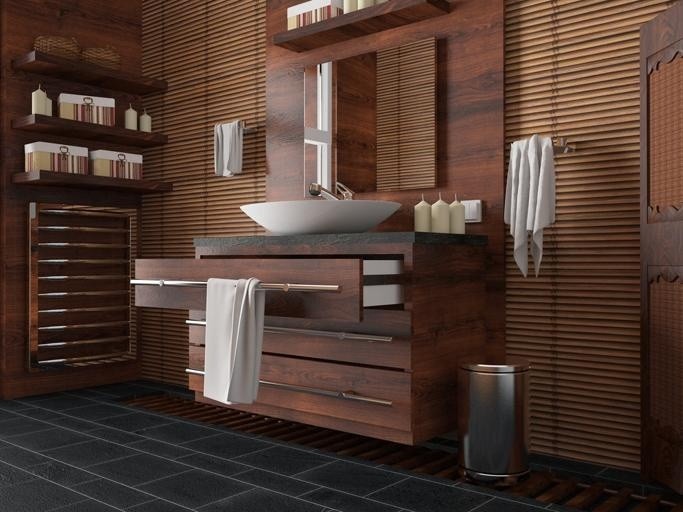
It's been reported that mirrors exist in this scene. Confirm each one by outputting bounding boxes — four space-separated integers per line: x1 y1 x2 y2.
304 37 436 193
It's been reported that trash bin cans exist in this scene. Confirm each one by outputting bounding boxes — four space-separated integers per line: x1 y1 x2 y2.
457 355 532 487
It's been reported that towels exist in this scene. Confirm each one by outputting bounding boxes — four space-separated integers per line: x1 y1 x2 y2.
213 120 244 178
503 135 556 279
202 277 265 407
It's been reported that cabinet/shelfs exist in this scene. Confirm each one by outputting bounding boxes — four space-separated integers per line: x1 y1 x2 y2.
133 232 503 447
10 50 175 192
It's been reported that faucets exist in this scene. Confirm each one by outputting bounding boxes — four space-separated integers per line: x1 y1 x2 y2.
308 181 355 200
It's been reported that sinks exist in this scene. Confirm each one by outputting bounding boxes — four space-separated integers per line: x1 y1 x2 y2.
239 199 403 234
